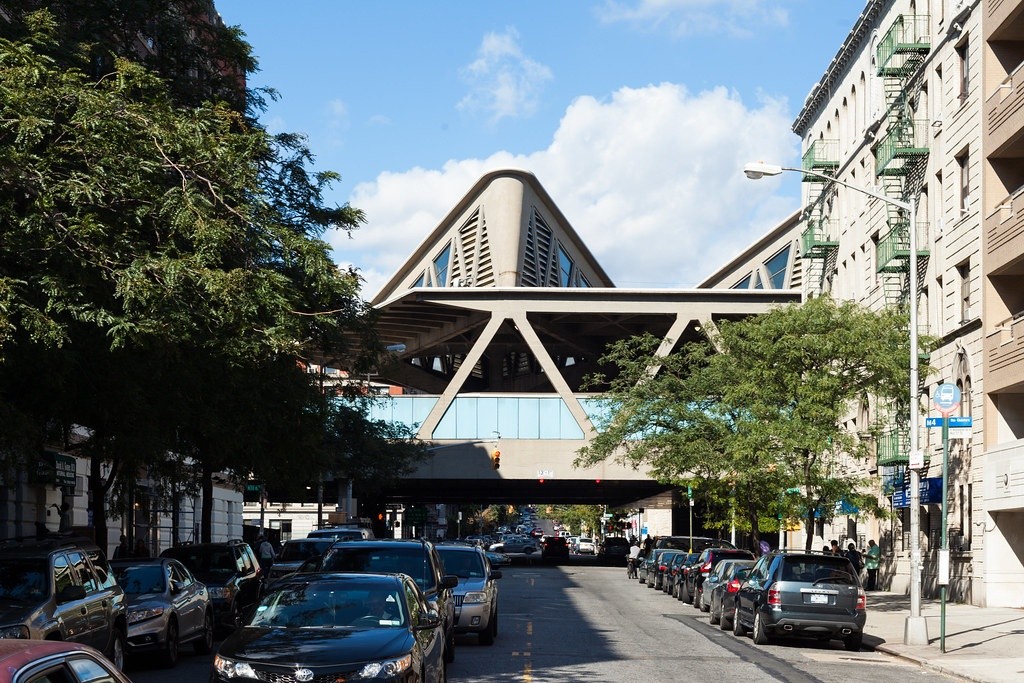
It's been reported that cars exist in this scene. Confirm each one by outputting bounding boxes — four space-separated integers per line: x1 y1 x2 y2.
0 638 133 683
638 535 756 608
489 509 569 558
599 537 629 559
434 544 503 645
108 558 216 667
699 559 755 612
441 535 511 570
709 562 757 631
209 571 447 683
269 539 338 586
552 519 595 555
307 528 375 543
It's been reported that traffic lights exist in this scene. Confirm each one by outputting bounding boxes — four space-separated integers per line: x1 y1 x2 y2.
491 450 500 470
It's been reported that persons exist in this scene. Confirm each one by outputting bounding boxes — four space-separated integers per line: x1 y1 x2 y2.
471 541 490 551
52 503 73 532
628 534 652 579
823 540 880 591
254 535 276 579
134 539 150 559
113 535 133 559
420 532 447 544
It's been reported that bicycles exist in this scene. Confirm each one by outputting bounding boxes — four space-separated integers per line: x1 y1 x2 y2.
625 554 634 579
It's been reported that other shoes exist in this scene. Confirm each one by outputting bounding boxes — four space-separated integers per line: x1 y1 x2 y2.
864 588 874 591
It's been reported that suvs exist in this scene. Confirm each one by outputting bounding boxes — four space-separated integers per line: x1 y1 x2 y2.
143 538 266 632
315 534 459 662
733 548 867 651
0 533 128 672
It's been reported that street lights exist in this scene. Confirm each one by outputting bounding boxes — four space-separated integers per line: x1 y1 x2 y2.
745 161 929 645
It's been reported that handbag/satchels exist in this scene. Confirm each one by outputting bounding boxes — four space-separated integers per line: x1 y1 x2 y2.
857 552 865 570
